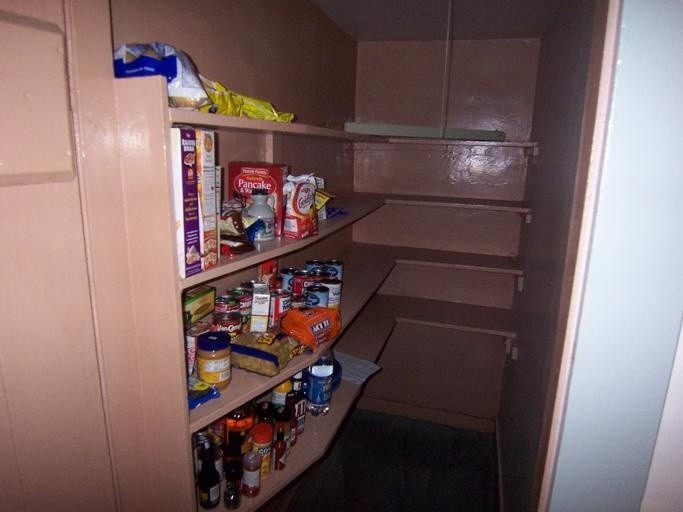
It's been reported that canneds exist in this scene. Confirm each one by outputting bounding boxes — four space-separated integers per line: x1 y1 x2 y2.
213 259 345 334
196 331 234 391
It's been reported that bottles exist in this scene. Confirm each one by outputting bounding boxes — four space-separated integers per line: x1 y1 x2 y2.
306 348 334 416
246 188 277 241
223 372 309 512
198 437 221 509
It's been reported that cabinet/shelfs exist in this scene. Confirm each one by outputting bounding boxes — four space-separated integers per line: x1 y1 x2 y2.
120 106 538 512
